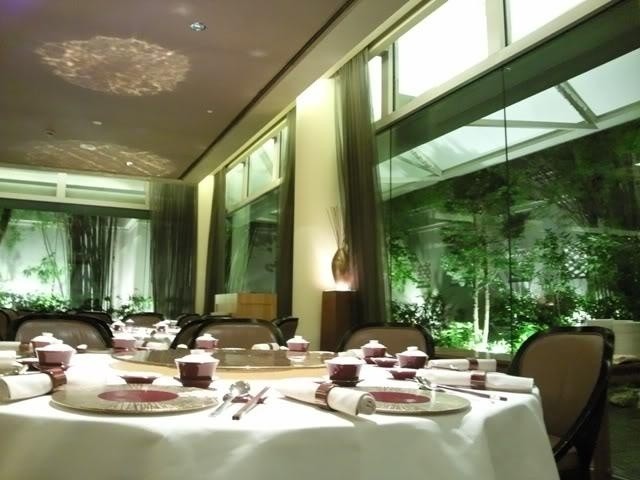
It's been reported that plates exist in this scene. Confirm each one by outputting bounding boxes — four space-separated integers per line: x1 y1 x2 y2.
32 363 73 373
120 375 159 384
338 385 471 415
372 357 397 366
51 383 223 417
173 376 219 389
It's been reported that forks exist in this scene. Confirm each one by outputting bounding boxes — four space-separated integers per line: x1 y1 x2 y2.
415 375 445 393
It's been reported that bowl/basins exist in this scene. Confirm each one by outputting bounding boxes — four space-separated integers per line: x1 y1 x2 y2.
386 369 416 380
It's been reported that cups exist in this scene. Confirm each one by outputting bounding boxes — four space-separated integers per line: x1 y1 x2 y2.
30 332 77 365
174 349 221 380
111 332 137 348
397 345 427 368
194 333 219 349
111 318 178 333
286 335 311 351
360 340 387 364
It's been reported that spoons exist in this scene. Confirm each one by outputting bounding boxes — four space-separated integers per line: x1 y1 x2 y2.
210 381 250 418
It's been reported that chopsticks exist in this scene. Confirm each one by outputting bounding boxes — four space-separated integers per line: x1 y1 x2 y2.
232 384 272 419
438 385 508 401
